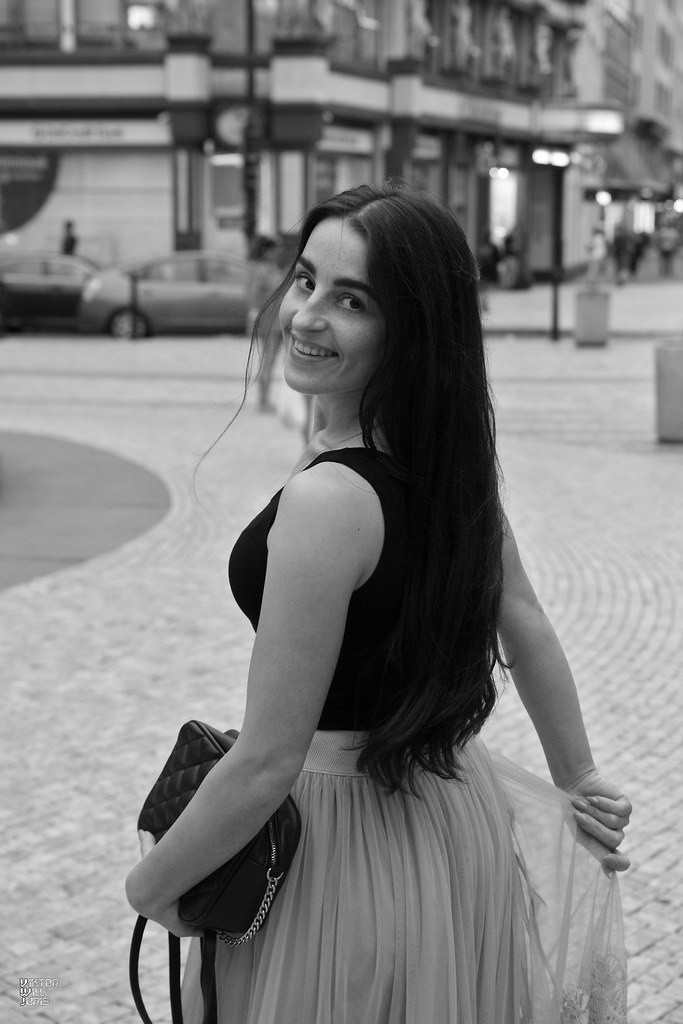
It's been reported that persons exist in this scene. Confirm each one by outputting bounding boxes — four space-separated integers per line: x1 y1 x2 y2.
57 219 78 255
126 181 641 1024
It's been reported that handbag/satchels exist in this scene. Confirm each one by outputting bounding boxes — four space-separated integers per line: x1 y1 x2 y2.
138 718 301 948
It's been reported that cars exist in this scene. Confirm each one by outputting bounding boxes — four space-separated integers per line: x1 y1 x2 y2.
80 251 286 340
1 251 106 328
